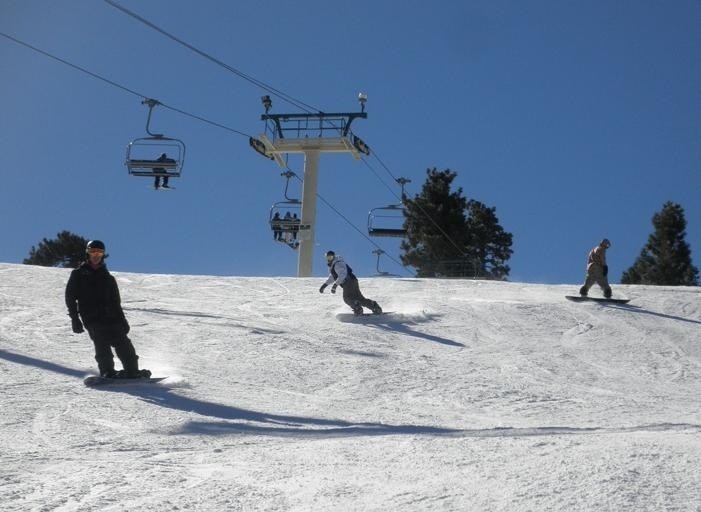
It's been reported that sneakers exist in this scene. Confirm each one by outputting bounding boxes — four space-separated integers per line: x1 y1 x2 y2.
354 308 363 315
371 301 382 314
102 370 151 378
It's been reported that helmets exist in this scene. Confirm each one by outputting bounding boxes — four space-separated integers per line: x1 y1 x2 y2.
85 240 105 260
327 251 334 256
600 239 611 249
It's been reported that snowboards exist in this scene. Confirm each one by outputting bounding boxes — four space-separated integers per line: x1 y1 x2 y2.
565 296 630 304
336 312 403 325
84 374 167 386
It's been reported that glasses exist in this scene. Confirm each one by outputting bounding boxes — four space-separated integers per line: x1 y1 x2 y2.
88 249 104 256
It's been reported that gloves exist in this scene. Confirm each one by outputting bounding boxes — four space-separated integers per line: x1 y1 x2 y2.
331 284 337 293
603 265 608 276
123 318 129 334
320 284 328 293
72 319 84 333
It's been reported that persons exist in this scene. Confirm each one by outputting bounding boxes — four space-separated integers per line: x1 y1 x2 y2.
151 151 178 190
578 237 614 300
282 211 294 242
318 247 386 318
293 214 301 241
64 238 151 386
273 212 284 242
